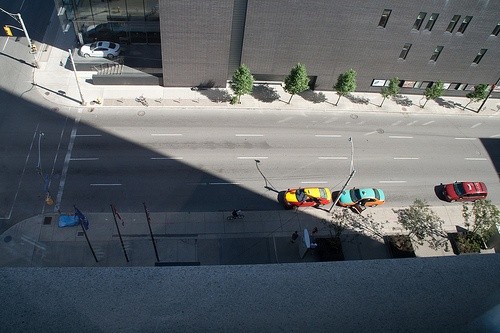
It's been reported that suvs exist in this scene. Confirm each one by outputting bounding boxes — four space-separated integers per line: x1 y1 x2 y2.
442 181 488 203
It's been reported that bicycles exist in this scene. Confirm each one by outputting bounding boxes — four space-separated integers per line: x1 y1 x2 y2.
226 210 245 222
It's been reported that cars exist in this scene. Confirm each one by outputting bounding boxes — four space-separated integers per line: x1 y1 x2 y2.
80 41 121 59
336 187 385 208
282 187 332 209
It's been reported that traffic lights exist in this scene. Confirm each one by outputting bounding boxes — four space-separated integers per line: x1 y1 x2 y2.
4 27 12 36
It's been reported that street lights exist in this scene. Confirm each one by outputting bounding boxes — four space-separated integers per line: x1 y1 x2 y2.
328 137 357 214
34 132 61 214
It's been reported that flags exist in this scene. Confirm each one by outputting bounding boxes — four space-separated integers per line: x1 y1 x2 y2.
77 209 89 230
47 194 54 206
113 208 124 227
145 206 151 221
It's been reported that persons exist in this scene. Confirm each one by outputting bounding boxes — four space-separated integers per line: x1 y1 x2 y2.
232 209 239 219
312 226 318 237
291 231 299 244
